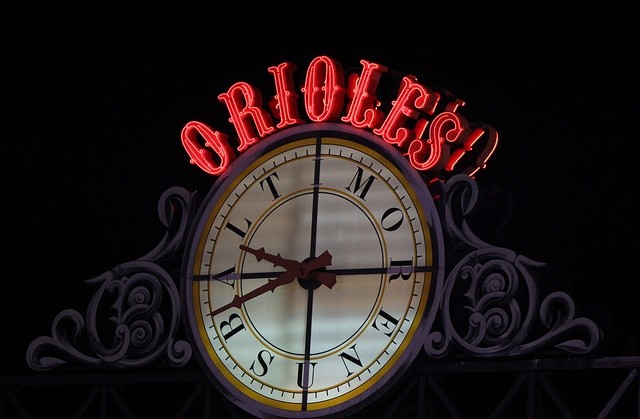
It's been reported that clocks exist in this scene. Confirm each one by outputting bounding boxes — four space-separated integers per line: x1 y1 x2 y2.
183 122 445 419
